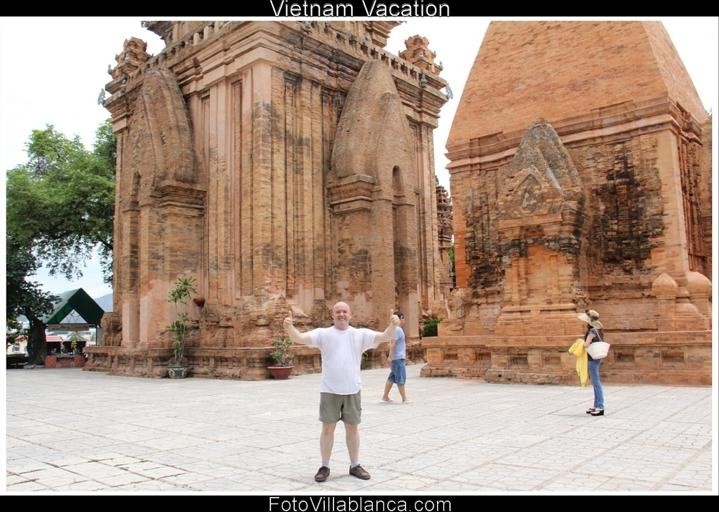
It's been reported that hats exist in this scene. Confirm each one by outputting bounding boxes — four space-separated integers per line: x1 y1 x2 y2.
578 310 604 329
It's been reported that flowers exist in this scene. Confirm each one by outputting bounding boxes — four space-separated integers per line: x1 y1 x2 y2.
265 329 296 366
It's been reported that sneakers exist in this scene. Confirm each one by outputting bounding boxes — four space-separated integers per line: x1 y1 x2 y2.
403 400 414 404
314 466 330 482
381 399 393 404
350 464 370 479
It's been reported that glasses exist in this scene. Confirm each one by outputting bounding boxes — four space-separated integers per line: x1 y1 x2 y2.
401 317 404 320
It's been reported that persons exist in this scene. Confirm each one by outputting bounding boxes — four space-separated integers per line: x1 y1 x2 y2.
283 302 402 482
577 310 604 416
380 311 414 405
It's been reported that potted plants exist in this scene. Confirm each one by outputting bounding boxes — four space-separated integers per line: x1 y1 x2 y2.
164 275 201 378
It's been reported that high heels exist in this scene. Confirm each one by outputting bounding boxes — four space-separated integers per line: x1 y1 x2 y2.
586 408 595 414
591 410 604 415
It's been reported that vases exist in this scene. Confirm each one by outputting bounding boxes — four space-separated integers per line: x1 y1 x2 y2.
267 366 293 378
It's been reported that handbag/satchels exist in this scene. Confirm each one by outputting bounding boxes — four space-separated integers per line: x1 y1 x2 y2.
585 342 610 359
